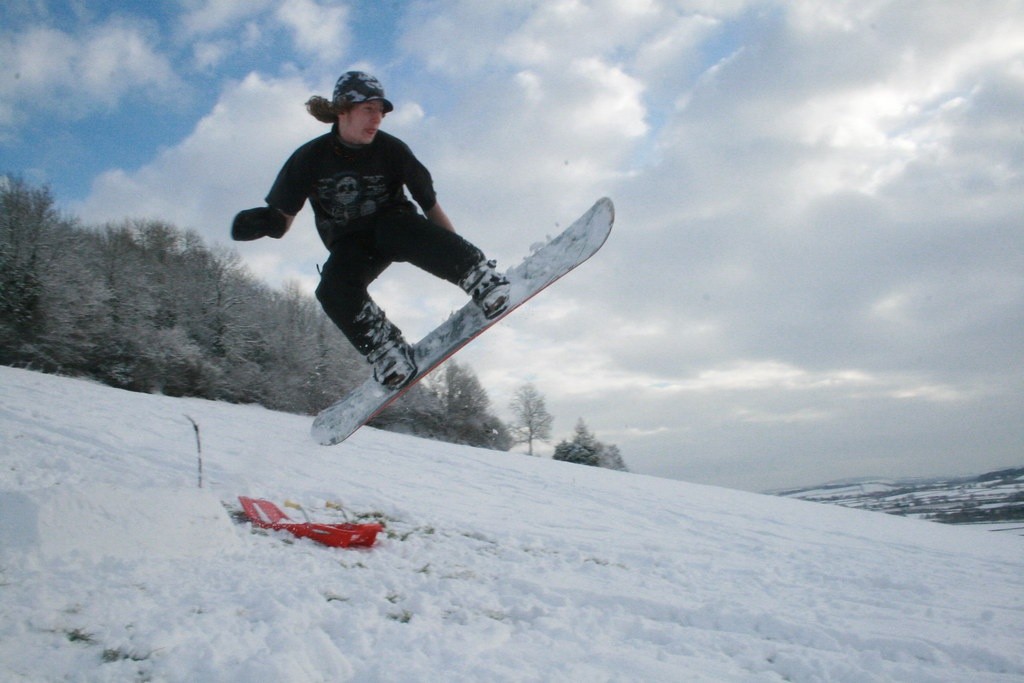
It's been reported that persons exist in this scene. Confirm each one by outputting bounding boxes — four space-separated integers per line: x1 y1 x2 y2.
231 68 512 392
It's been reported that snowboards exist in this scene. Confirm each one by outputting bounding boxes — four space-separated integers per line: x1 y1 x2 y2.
310 197 613 448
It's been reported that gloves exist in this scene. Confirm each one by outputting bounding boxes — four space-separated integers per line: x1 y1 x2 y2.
232 205 288 241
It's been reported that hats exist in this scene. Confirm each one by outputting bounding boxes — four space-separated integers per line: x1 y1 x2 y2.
333 71 393 113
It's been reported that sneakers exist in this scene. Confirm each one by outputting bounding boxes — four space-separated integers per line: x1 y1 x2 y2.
367 338 417 390
459 260 510 319
